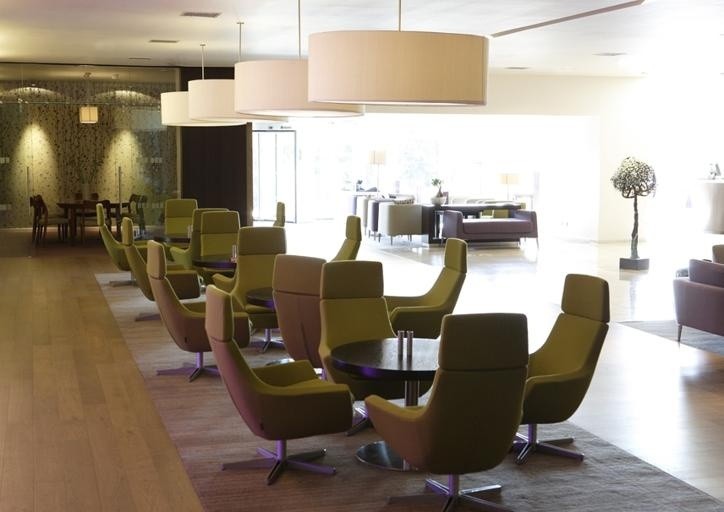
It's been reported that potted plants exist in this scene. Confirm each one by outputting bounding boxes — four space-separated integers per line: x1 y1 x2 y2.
429 178 446 205
610 156 657 270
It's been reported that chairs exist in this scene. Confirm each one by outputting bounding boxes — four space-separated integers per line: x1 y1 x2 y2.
29 192 150 247
673 244 723 344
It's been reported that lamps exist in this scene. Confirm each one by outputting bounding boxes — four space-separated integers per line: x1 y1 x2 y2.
78 72 97 125
